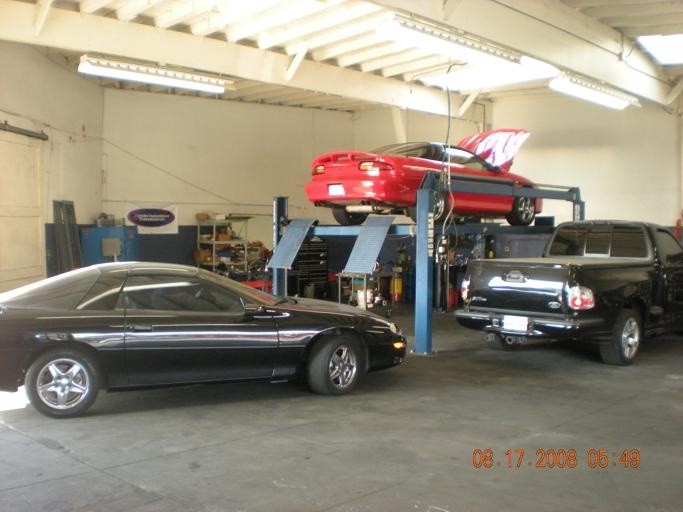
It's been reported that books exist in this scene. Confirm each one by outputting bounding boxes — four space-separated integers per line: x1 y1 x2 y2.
207 213 225 220
219 256 231 263
228 213 253 219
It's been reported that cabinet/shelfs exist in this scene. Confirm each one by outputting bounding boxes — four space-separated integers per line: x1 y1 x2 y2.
196 221 249 274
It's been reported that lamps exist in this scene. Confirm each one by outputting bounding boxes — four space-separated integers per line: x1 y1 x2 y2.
78 55 234 95
551 72 638 114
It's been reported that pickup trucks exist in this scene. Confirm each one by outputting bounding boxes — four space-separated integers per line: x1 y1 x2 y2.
454 220 683 366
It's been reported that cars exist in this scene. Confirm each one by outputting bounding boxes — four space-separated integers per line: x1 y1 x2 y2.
0 262 407 419
305 129 543 224
